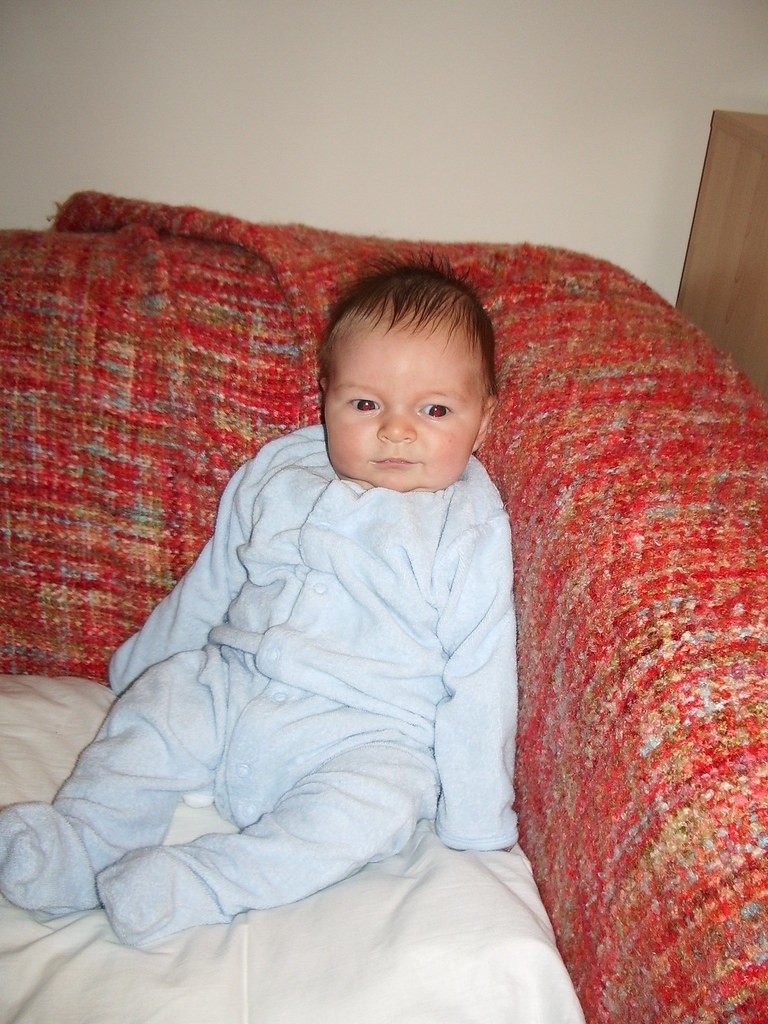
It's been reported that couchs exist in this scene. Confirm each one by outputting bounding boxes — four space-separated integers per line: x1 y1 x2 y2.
0 190 768 1024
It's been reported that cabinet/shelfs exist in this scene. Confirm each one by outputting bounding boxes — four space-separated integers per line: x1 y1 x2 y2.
676 109 768 399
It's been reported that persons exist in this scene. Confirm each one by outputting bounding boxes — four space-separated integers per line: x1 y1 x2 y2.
0 254 518 944
